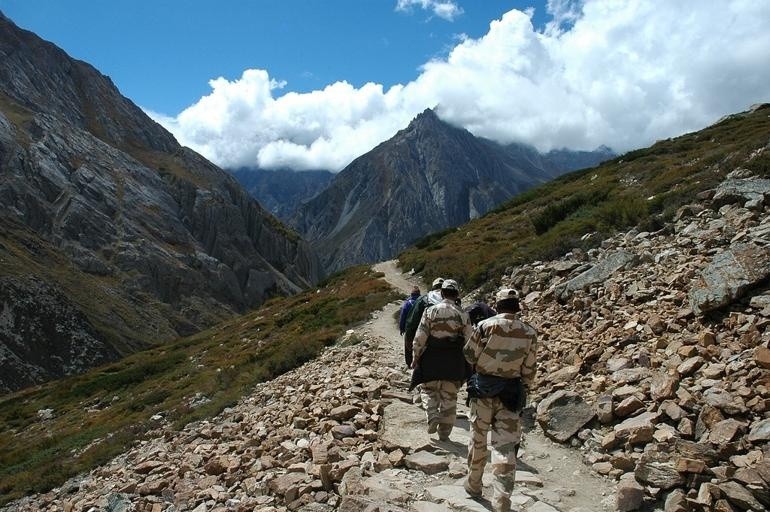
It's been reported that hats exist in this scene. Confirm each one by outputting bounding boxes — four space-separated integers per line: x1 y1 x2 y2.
442 279 458 291
432 278 444 286
496 289 519 302
411 286 420 294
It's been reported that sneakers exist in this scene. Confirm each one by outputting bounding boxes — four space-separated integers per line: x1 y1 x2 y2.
463 479 483 499
427 422 449 441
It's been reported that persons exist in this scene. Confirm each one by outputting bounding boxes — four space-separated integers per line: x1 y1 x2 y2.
408 279 472 442
462 286 539 511
465 292 498 408
404 277 446 353
399 285 421 370
